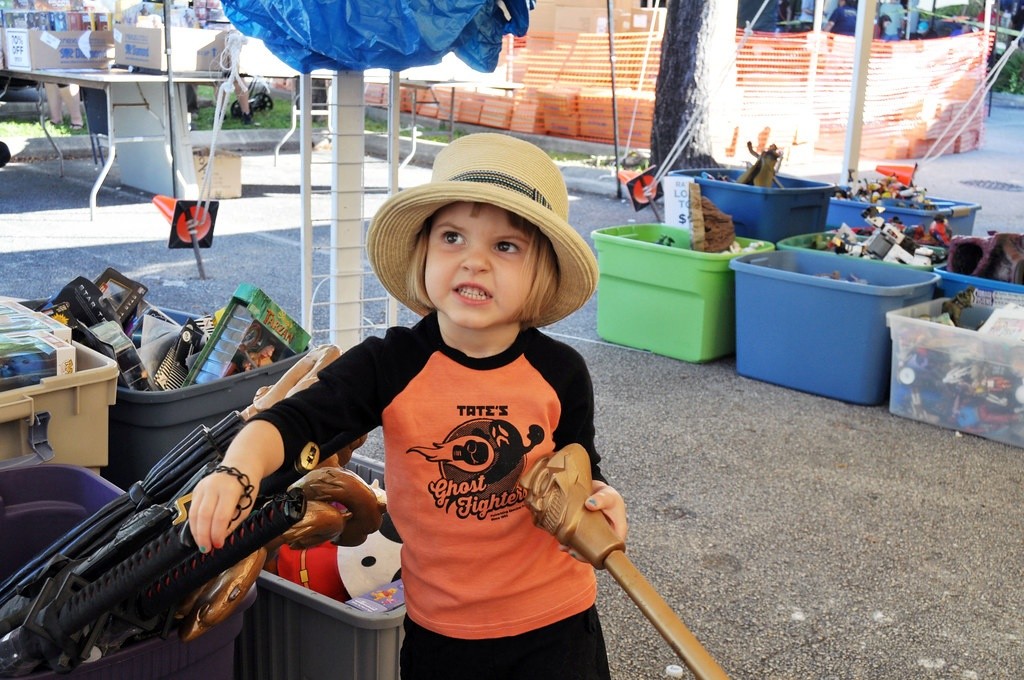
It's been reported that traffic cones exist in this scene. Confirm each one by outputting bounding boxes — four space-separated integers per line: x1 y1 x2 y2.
152 195 220 249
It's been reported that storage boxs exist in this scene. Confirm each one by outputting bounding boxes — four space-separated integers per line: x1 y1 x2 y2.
192 145 243 198
777 231 949 298
0 463 258 680
669 167 836 245
589 223 775 363
0 300 77 393
113 23 229 73
0 340 122 474
2 28 114 70
100 344 312 493
76 319 158 392
727 250 942 404
232 452 407 680
934 263 1024 327
825 185 982 235
180 283 313 385
884 297 1024 449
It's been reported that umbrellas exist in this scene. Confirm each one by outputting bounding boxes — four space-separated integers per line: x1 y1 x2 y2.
0 345 341 604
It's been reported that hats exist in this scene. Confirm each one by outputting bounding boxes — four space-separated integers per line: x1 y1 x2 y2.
365 133 599 328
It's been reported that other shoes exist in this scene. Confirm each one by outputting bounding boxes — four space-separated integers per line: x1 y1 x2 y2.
212 112 226 122
240 111 253 124
192 111 200 120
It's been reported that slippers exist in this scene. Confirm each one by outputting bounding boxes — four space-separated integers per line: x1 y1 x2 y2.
70 127 84 131
50 121 64 125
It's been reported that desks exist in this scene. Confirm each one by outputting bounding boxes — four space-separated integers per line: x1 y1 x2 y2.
0 68 273 229
275 75 526 180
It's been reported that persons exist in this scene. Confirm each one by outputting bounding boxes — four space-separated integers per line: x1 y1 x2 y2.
188 130 625 680
44 83 86 130
214 80 254 124
184 83 199 121
776 0 1024 50
